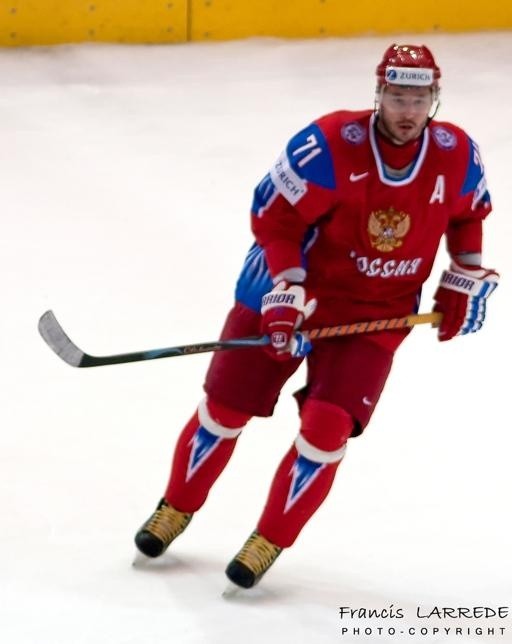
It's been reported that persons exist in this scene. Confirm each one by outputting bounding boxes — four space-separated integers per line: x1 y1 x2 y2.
135 43 499 589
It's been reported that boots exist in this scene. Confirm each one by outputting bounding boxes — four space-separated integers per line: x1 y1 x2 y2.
225 530 283 589
134 497 194 558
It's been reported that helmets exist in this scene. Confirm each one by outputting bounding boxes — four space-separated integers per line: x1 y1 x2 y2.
376 44 441 88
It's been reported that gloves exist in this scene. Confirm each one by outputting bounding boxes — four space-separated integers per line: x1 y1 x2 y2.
259 280 312 363
432 256 501 341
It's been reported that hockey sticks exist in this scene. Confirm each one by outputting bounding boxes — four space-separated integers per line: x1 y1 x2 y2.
37 310 446 368
